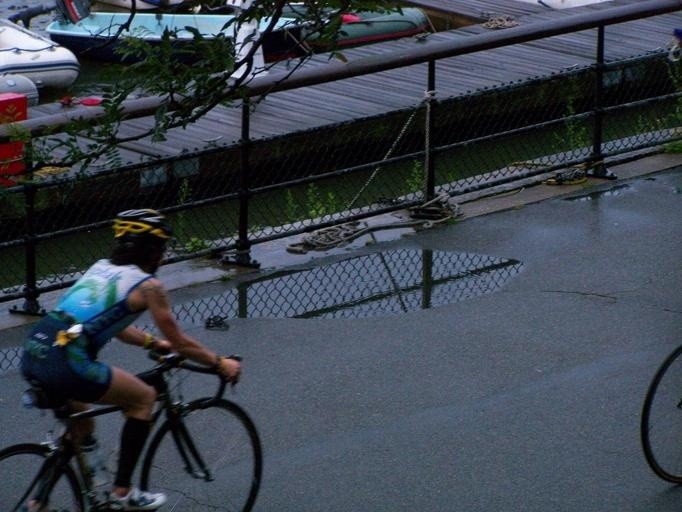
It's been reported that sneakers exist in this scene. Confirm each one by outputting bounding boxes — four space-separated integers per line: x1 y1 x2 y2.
81 470 166 512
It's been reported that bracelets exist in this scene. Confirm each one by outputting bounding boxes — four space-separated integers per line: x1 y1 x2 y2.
142 333 155 350
213 354 226 373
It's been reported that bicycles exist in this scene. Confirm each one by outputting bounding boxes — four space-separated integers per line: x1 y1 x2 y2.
0 347 263 512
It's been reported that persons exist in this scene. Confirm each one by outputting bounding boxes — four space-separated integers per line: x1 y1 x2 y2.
18 208 243 512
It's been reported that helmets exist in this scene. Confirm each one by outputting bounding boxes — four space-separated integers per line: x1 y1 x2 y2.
114 208 172 244
